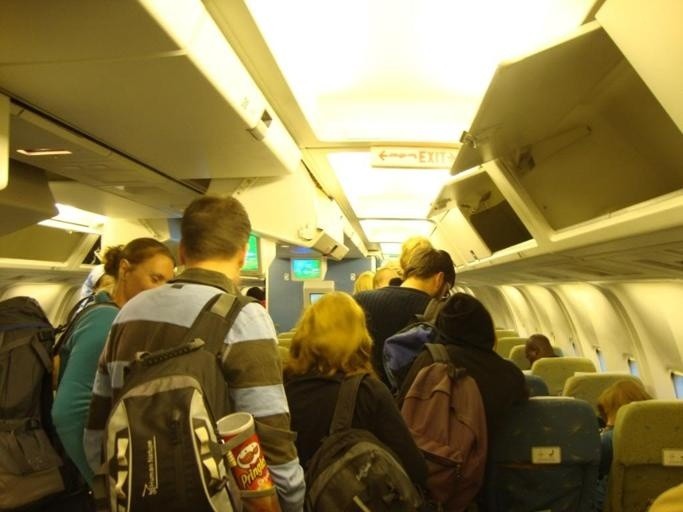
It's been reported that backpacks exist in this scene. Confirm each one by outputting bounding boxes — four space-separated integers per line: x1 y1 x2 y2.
303 427 437 512
399 345 488 510
381 296 447 393
0 294 119 512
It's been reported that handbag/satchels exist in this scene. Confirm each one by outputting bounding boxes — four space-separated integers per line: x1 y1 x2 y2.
104 292 274 512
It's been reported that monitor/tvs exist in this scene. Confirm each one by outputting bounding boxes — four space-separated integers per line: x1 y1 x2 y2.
290 255 328 281
239 232 276 279
310 292 325 305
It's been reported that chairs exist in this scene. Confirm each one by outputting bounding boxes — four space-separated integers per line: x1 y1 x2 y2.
486 330 683 512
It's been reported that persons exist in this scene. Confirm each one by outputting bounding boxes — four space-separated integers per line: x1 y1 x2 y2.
525 335 558 368
280 237 525 510
597 379 653 479
48 196 305 512
